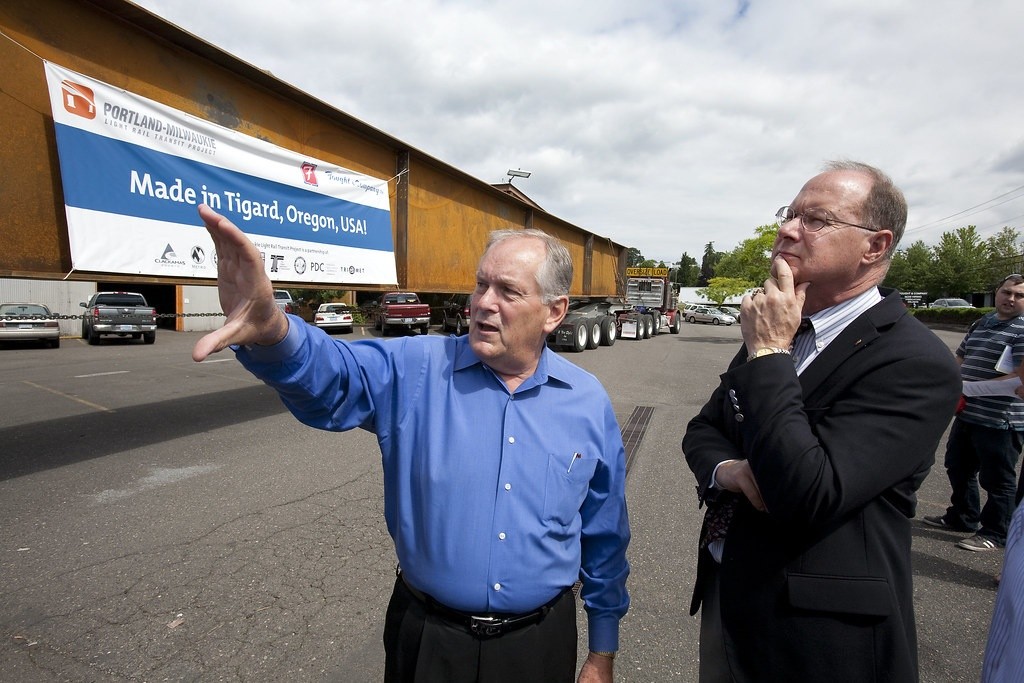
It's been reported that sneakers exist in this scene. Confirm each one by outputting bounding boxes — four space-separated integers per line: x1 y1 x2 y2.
923 515 978 533
957 533 1000 550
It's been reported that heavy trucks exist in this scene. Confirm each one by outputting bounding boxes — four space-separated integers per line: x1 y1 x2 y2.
544 266 681 353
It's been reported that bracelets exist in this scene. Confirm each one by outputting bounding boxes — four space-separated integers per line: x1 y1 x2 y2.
590 650 616 659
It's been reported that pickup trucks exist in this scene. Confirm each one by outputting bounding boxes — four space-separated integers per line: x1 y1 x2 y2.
79 292 157 344
373 293 430 336
273 290 303 314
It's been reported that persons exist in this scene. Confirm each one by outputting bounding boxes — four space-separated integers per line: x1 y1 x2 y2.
976 365 1023 682
924 273 1024 552
191 204 632 683
682 160 962 683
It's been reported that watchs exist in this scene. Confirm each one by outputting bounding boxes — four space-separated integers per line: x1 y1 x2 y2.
746 347 790 363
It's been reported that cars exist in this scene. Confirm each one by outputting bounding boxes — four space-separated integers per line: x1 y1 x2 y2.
0 302 61 349
905 302 933 308
930 299 977 309
312 303 354 333
716 307 740 322
685 308 736 326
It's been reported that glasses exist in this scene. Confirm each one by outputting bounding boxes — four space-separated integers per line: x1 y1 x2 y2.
1004 273 1024 281
774 206 876 235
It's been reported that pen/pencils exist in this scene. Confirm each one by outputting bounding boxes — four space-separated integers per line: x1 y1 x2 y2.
568 452 582 473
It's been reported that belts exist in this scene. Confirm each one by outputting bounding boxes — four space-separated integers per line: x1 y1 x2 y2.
396 572 575 637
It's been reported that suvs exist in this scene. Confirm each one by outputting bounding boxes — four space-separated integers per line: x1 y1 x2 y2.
442 293 471 336
682 304 710 317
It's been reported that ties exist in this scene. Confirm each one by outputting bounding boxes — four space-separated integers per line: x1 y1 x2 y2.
699 317 812 551
